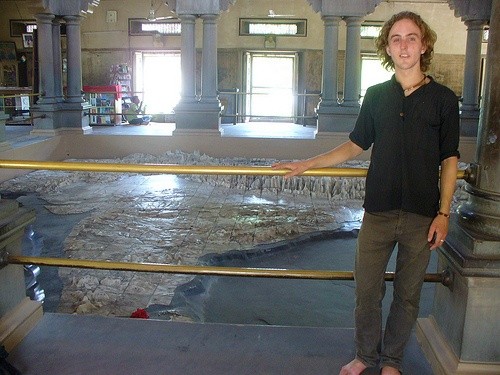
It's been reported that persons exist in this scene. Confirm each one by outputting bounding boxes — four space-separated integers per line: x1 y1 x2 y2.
126 96 149 125
271 11 460 375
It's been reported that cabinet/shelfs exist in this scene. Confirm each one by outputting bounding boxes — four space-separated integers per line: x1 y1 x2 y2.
82 85 122 126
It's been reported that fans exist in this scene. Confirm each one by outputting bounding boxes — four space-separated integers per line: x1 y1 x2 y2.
248 0 296 18
132 0 177 23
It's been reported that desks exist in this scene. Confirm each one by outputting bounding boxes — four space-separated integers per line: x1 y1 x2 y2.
218 88 240 125
302 88 343 126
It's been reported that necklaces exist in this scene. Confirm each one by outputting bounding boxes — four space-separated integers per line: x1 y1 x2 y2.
403 72 426 92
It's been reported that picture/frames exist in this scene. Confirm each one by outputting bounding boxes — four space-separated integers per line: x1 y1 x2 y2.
21 33 34 49
0 41 18 61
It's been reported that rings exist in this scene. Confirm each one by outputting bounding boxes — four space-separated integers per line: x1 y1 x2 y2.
440 240 444 242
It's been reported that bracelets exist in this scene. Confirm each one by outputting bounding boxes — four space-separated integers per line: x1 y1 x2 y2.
438 211 451 219
